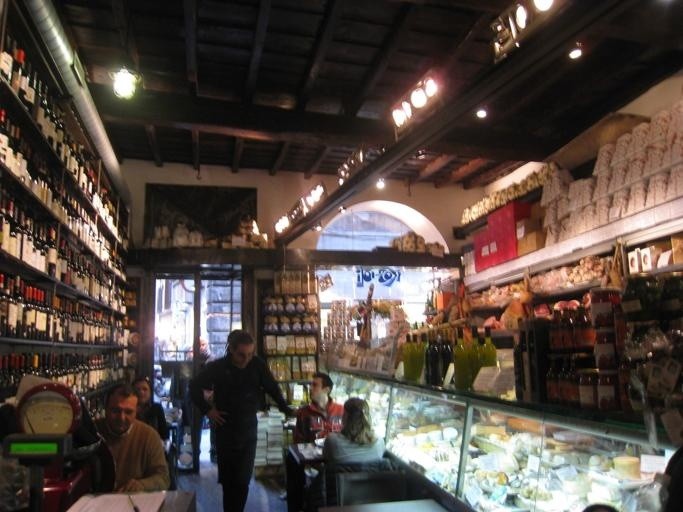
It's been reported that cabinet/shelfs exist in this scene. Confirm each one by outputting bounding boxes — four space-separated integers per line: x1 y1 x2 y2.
0 1 140 433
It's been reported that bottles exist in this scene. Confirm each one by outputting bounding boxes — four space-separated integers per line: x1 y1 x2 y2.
513 330 532 402
0 32 129 419
545 356 579 408
547 306 596 355
401 324 498 392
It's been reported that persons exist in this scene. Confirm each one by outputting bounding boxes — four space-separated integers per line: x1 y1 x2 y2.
153 368 170 405
189 331 293 511
87 383 172 495
130 379 168 442
295 371 344 440
197 335 215 364
322 396 384 464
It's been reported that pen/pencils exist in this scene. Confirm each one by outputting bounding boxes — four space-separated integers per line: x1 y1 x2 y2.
128 496 140 512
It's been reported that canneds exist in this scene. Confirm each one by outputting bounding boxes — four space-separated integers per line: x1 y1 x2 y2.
577 263 683 412
